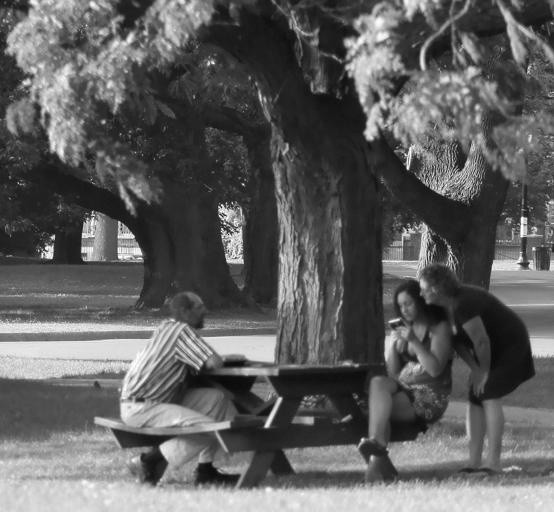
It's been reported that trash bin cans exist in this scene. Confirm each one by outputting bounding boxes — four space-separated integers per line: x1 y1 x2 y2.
531 246 550 271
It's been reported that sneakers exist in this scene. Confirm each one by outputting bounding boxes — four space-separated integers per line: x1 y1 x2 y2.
126 454 154 486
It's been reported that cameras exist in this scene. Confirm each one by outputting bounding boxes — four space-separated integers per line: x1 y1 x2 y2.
388 319 407 331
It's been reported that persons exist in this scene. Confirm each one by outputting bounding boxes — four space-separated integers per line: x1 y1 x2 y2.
356 280 459 481
420 264 535 477
119 292 242 486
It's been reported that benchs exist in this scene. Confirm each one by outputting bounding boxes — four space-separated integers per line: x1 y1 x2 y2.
93 414 269 436
294 407 434 432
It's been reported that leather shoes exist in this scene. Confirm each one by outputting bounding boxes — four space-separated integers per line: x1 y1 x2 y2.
193 469 241 487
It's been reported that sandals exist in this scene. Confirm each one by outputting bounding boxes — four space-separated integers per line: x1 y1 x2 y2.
358 438 388 458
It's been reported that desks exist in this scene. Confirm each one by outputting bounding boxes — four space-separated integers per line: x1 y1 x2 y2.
189 365 399 490
